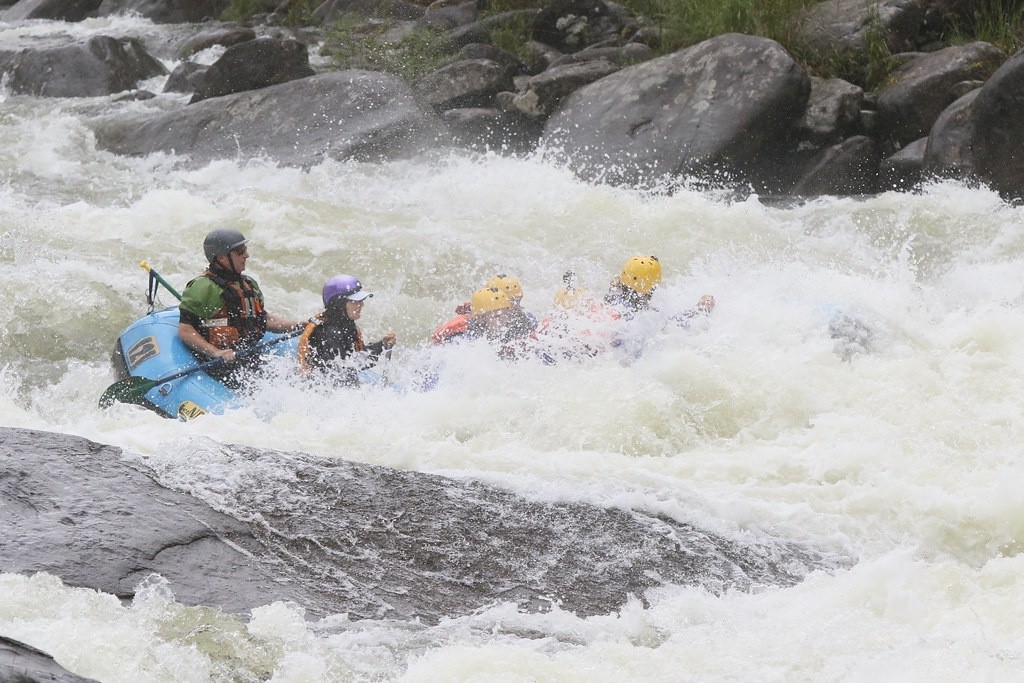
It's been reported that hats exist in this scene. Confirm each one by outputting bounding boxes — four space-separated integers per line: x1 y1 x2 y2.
344 291 373 304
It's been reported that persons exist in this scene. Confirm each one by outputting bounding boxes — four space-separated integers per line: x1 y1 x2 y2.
296 274 396 387
433 256 714 357
178 227 311 391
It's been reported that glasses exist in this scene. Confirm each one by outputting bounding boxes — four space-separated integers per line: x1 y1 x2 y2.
233 246 247 254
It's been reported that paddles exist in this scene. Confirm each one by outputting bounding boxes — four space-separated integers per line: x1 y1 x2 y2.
98 316 310 415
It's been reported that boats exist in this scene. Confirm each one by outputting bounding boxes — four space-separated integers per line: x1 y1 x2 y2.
112 305 304 421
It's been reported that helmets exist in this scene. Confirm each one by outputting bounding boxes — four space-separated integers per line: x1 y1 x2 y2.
620 256 662 293
203 229 248 265
321 274 361 309
470 288 510 317
485 274 523 299
